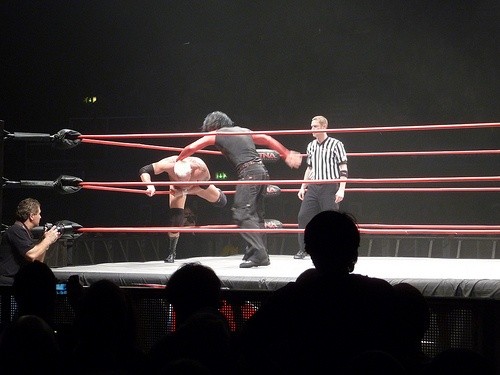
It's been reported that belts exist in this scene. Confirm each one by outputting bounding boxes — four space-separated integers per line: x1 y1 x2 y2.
237 160 263 170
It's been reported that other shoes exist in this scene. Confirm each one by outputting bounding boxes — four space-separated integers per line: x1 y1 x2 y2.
163 248 178 264
293 249 308 260
238 255 270 269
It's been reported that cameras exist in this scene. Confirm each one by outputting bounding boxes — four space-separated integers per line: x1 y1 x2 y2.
56 284 68 297
44 222 72 239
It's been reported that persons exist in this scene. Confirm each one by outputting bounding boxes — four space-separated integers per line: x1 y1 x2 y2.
176 207 201 260
0 198 63 337
295 210 393 374
294 116 348 259
174 111 302 268
139 155 227 263
0 262 489 375
